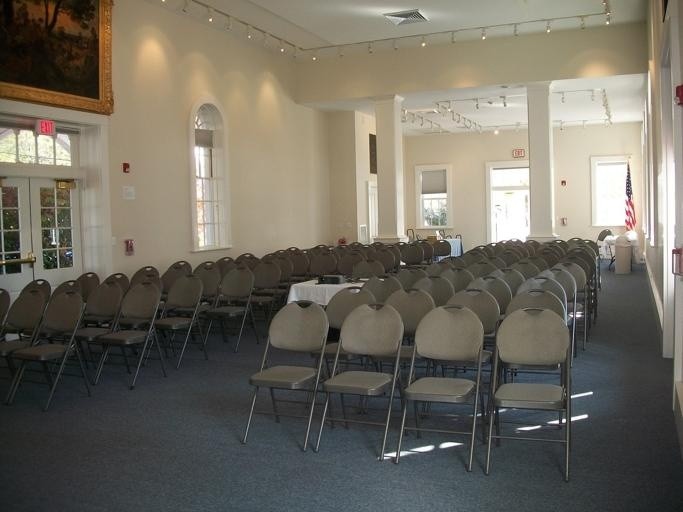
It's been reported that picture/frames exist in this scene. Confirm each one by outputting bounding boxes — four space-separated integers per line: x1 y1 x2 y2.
0 0 115 115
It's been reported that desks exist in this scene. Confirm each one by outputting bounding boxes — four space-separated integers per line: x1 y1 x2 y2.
606 241 640 271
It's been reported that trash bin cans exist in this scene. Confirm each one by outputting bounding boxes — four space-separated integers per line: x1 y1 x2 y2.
614 236 633 275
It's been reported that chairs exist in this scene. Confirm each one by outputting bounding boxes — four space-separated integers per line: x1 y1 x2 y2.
596 229 612 261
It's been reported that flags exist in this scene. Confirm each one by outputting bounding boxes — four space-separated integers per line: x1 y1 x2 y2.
625 161 637 231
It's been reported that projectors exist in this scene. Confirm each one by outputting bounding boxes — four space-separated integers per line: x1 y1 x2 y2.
318 274 344 283
